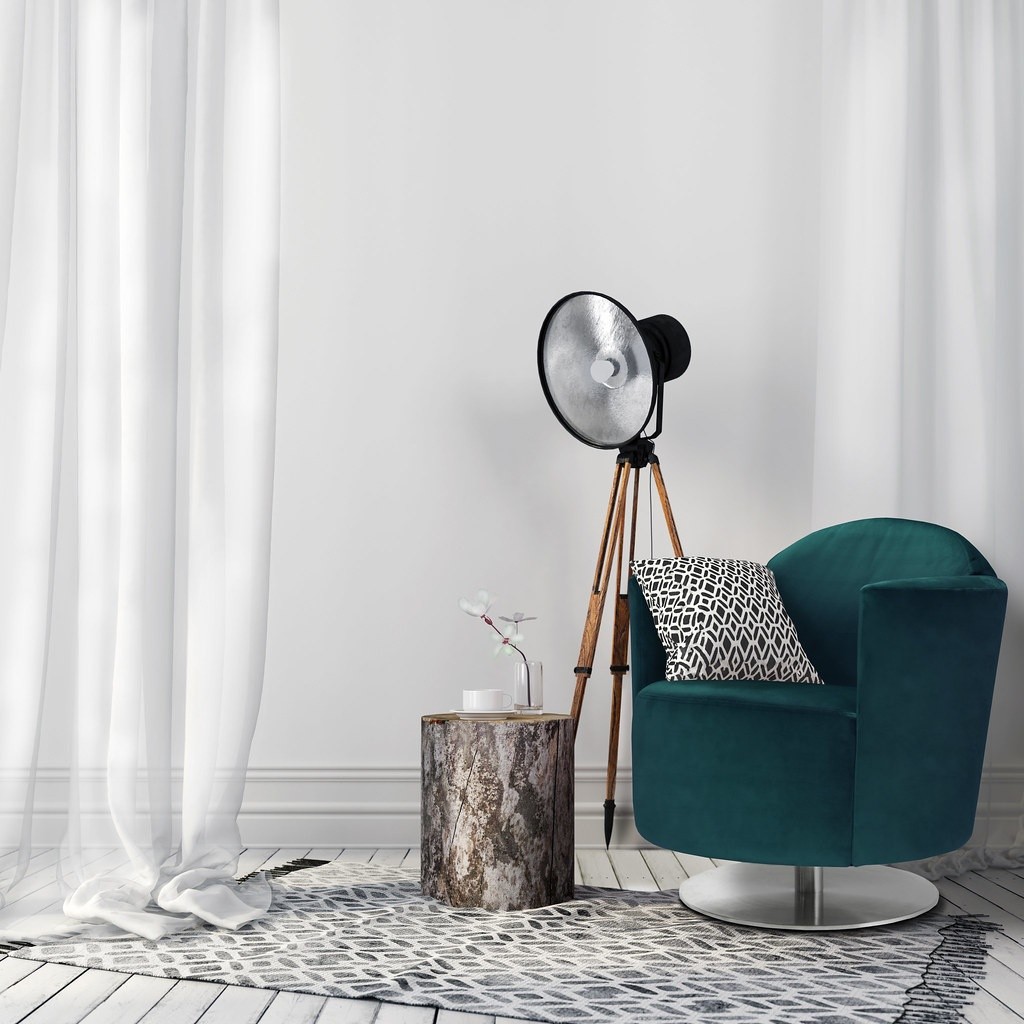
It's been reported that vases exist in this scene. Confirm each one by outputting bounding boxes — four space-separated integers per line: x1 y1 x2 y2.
514 658 546 716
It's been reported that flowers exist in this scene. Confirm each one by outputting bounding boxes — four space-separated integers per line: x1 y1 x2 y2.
459 589 539 706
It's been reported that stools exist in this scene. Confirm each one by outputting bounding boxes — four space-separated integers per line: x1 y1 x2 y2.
420 713 576 911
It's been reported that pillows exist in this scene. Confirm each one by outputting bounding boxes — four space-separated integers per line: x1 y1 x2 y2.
629 558 826 686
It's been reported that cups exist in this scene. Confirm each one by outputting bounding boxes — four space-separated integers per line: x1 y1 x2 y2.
463 689 513 711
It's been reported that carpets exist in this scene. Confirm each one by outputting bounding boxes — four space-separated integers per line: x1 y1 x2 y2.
0 857 1004 1024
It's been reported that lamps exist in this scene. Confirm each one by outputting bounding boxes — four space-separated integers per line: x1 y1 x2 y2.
536 291 694 468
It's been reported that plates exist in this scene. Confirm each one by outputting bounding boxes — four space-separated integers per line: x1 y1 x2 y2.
451 708 517 720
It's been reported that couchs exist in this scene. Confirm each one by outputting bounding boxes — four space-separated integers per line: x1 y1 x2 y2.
626 509 1012 937
